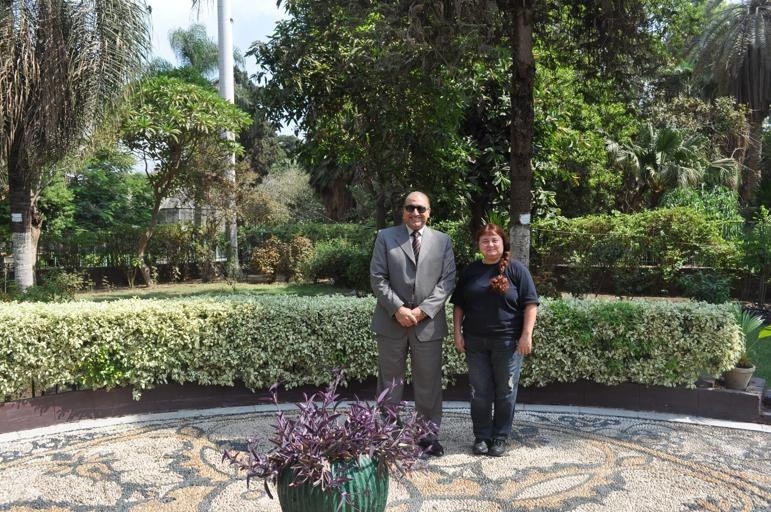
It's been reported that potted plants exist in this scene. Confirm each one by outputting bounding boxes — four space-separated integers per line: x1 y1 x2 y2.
208 364 442 512
720 296 771 391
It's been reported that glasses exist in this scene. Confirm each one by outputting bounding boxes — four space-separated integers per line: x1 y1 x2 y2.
403 204 431 214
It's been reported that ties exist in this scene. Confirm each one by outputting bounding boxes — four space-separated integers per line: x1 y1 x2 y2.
412 231 422 264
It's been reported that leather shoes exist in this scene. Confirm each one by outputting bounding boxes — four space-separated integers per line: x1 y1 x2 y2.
418 439 445 457
489 437 507 456
474 437 492 455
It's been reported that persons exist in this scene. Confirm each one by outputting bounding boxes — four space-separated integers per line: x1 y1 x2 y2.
449 221 542 457
367 191 459 458
